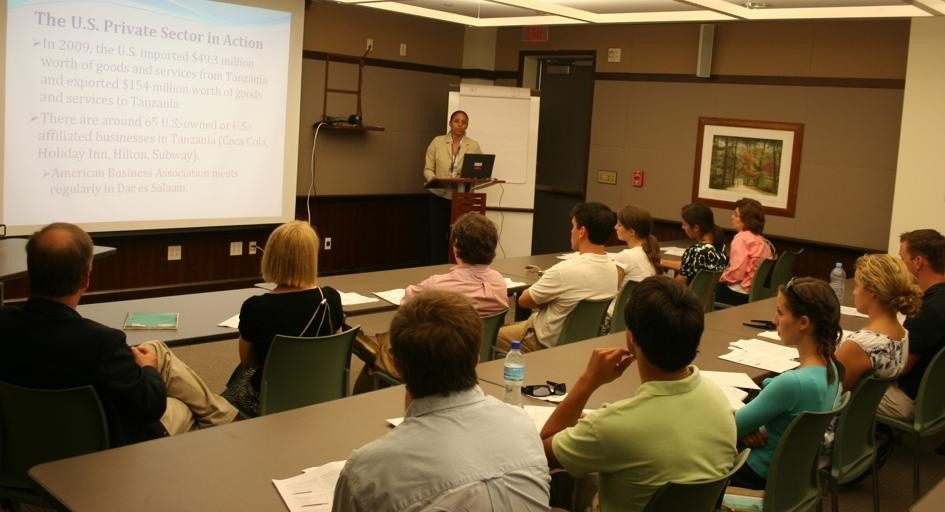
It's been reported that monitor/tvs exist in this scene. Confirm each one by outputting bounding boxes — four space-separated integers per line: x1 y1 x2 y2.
460 154 495 177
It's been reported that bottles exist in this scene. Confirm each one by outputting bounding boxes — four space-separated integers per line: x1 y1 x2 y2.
504 341 525 408
830 263 847 304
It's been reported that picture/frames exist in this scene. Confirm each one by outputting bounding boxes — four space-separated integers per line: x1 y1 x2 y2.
692 117 804 218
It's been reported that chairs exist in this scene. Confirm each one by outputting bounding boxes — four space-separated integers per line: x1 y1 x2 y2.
1 384 110 511
714 258 777 309
688 269 723 314
874 348 944 502
817 377 892 510
771 250 804 297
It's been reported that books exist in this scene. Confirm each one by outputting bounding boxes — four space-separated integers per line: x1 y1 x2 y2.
122 309 180 330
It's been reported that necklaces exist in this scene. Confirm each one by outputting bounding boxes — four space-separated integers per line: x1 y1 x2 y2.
794 355 820 370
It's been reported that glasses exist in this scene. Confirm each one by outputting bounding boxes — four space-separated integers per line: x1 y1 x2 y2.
522 381 566 396
787 277 800 301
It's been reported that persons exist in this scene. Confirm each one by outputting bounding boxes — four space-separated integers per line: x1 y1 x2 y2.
495 199 621 356
600 204 666 334
423 110 486 262
871 227 945 430
218 219 346 418
663 201 731 306
349 210 511 397
720 197 777 300
730 274 846 511
0 220 242 452
816 251 924 470
327 290 554 511
538 277 739 511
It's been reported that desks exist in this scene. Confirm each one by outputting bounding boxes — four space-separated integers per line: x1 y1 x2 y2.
74 238 696 366
27 279 871 511
